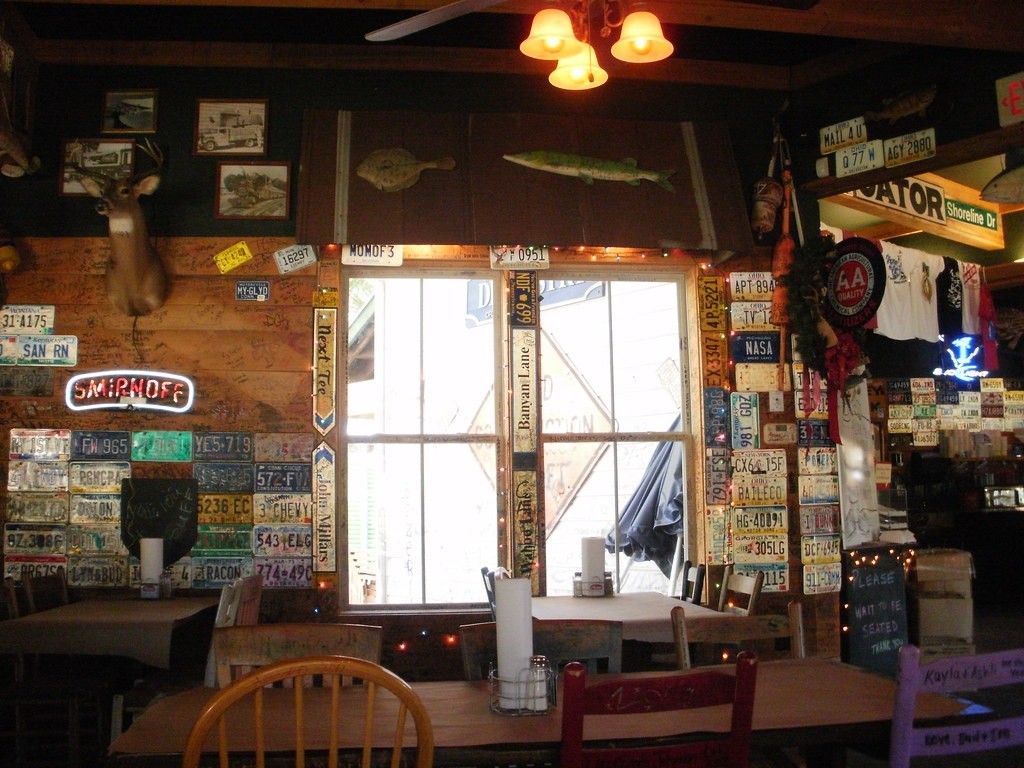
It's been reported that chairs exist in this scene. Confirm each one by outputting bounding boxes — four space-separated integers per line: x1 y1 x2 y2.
0 563 1024 768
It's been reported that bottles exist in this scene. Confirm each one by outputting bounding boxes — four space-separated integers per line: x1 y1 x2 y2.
573 572 582 597
604 572 613 596
525 655 551 711
163 570 171 598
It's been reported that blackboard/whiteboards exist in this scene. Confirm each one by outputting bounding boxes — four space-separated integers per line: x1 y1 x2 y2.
840 545 909 682
119 478 198 569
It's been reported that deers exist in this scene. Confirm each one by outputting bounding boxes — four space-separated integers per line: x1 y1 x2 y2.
59 137 170 314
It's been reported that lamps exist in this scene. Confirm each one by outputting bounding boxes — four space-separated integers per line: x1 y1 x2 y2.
520 1 674 91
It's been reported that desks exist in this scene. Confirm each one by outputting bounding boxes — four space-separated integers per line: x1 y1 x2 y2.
1 590 215 756
514 593 724 672
113 655 968 768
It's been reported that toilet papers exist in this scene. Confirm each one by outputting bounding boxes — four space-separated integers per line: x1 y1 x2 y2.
495 576 534 711
140 537 163 598
581 535 604 596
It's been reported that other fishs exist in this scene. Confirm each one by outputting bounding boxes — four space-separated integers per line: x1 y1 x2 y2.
503 152 676 193
356 149 456 191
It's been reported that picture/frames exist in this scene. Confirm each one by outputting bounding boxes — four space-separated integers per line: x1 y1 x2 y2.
194 97 270 155
214 159 292 221
101 86 160 133
60 139 135 198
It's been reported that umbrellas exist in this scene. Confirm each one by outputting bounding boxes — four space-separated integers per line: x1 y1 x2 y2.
606 409 684 596
